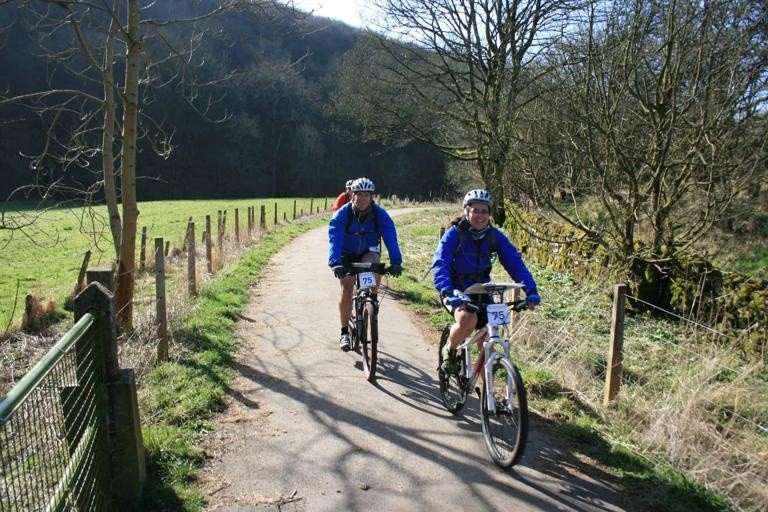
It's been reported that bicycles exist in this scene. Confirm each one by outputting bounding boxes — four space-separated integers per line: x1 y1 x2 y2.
344 262 391 379
434 298 530 469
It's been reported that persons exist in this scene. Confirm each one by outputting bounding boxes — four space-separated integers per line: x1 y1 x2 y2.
329 177 403 352
431 189 542 414
333 180 354 215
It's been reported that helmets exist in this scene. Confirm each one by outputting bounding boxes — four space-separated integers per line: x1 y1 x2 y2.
345 177 376 192
462 189 494 209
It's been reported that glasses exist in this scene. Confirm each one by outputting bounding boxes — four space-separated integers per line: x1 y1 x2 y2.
472 207 488 215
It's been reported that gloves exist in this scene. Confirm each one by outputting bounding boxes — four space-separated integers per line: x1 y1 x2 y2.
332 264 346 279
445 294 464 309
526 293 542 305
390 263 403 278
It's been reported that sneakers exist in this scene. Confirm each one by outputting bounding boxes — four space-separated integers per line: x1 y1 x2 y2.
441 343 458 375
339 333 351 349
